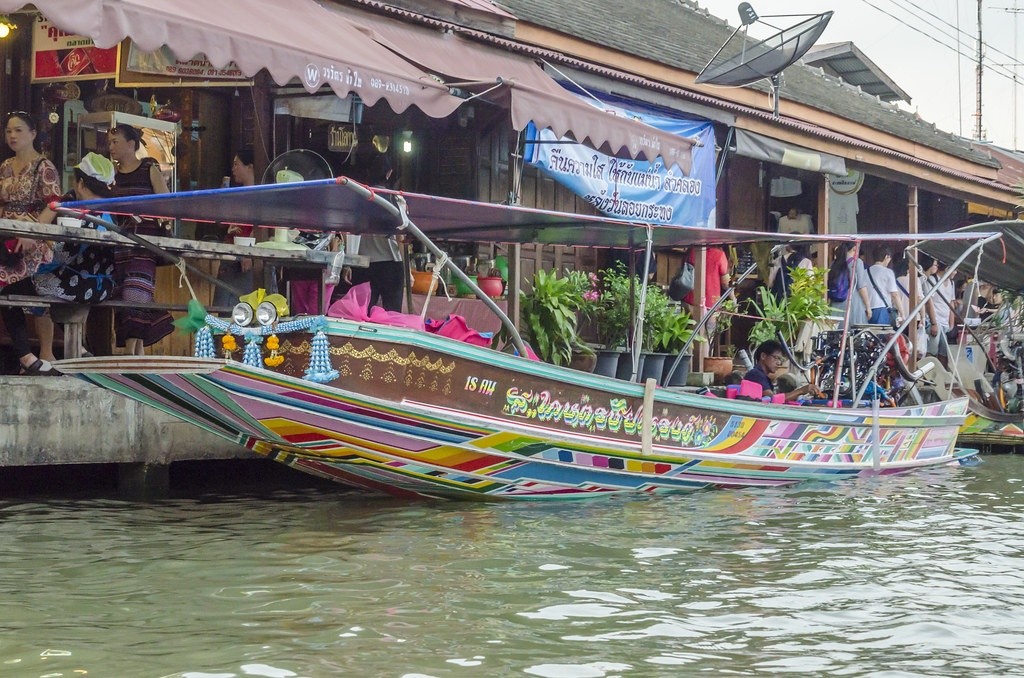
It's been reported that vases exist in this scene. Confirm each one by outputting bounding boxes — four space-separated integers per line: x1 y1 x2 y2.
452 276 478 296
477 277 503 296
561 350 598 374
411 272 440 296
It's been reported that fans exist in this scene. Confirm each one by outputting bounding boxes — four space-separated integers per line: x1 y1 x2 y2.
254 149 333 250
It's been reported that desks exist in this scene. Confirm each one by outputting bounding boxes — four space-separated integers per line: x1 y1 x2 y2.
957 324 997 345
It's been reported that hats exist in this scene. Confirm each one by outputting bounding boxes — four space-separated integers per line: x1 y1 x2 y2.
71 152 115 185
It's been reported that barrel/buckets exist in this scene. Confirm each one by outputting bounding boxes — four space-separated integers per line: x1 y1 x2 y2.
496 256 509 281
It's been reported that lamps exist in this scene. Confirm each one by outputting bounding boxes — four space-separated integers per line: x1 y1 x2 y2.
0 14 11 38
232 302 256 327
254 301 278 327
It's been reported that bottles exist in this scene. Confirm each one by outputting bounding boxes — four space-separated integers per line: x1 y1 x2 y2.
324 245 345 284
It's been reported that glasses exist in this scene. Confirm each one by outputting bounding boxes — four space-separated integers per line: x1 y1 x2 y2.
768 353 785 363
7 110 32 120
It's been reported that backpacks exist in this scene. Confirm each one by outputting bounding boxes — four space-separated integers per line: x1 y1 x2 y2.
827 256 854 302
771 254 805 310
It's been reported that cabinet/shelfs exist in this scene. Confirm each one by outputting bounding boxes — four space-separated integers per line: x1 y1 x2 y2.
76 111 177 238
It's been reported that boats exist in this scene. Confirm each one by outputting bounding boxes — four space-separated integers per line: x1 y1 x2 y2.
48 175 1024 511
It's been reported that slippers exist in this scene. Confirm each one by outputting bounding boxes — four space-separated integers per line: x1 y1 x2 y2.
18 359 46 376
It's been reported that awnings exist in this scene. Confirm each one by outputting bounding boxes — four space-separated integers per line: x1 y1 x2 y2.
0 0 703 176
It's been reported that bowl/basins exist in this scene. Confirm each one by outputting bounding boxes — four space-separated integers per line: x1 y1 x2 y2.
451 276 478 294
478 277 503 296
412 270 438 295
234 237 255 247
57 218 81 228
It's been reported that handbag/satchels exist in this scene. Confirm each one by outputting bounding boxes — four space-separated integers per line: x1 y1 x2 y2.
155 221 179 267
916 323 931 359
888 307 904 330
669 250 695 301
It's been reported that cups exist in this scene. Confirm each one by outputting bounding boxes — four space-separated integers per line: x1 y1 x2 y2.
223 177 230 189
346 235 361 254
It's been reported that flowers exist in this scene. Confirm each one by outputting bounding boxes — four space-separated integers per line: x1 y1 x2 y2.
560 268 600 355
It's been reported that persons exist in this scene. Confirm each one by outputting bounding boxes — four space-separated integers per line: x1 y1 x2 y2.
212 146 254 318
828 240 872 328
715 339 819 405
0 110 176 376
684 244 736 357
859 245 1024 368
768 231 814 309
776 201 815 234
339 159 404 312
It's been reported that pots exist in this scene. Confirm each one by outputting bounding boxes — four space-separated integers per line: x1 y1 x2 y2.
416 255 495 276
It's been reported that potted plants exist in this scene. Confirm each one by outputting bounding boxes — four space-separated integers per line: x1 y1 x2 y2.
703 294 741 386
642 305 683 386
593 259 643 378
650 307 709 386
746 265 846 381
615 272 670 383
